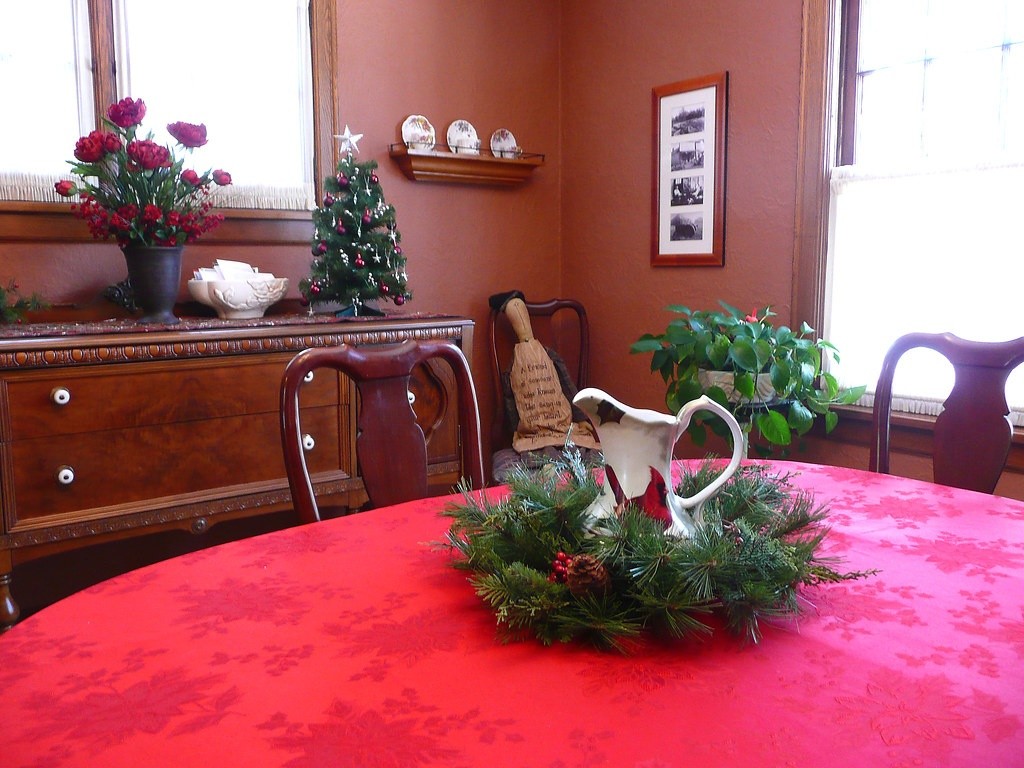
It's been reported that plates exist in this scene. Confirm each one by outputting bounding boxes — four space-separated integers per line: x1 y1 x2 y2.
446 119 481 155
401 114 436 150
490 128 518 159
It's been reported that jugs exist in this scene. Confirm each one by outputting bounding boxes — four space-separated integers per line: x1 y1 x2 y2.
572 387 744 549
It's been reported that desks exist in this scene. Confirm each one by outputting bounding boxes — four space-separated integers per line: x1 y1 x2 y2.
0 453 1024 768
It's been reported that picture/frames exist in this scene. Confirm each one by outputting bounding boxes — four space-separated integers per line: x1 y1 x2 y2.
651 69 726 268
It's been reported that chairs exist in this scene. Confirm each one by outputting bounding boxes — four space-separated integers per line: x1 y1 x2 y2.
489 299 604 483
279 339 485 525
867 332 1024 495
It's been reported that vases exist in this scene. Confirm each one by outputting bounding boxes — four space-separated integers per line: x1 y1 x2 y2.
699 363 777 405
115 247 184 325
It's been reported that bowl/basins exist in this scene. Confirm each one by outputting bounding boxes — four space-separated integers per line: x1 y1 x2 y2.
188 278 288 321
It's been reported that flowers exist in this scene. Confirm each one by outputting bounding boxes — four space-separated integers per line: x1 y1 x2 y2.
55 97 233 247
635 296 867 453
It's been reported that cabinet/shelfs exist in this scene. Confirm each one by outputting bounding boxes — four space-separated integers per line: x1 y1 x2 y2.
0 335 474 610
